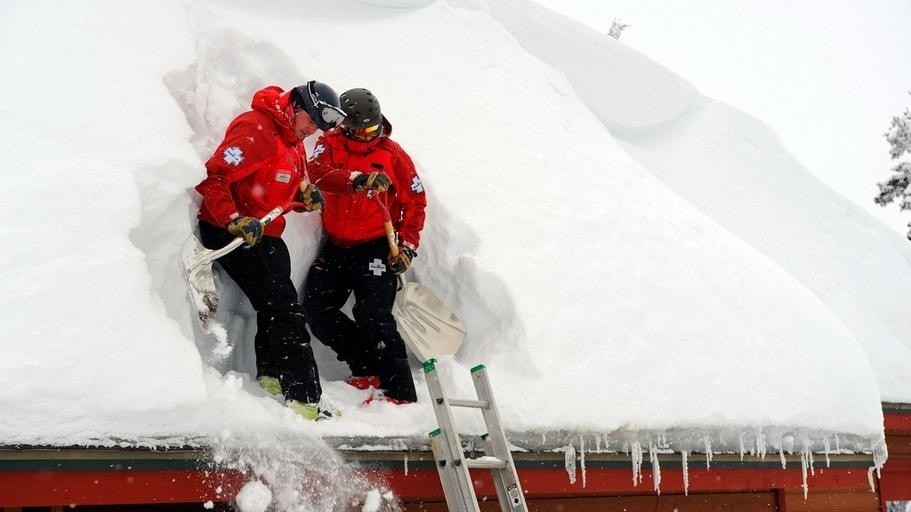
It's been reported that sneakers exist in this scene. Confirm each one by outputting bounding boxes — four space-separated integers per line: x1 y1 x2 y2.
345 377 417 406
259 374 342 422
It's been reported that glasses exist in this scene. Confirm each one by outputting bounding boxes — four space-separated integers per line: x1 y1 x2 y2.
346 126 380 139
307 81 348 125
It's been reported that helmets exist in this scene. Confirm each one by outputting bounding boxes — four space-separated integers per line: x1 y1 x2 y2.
336 89 381 140
290 83 339 132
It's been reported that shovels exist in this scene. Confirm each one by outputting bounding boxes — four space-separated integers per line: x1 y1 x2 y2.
181 176 305 336
372 185 468 364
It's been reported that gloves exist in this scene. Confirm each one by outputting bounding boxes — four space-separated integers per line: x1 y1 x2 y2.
301 180 322 210
351 170 397 196
386 242 414 276
227 217 264 247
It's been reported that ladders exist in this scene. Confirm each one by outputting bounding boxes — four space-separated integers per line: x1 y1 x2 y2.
423 358 529 512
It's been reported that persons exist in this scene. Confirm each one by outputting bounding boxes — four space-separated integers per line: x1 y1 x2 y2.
302 87 428 407
193 80 347 423
